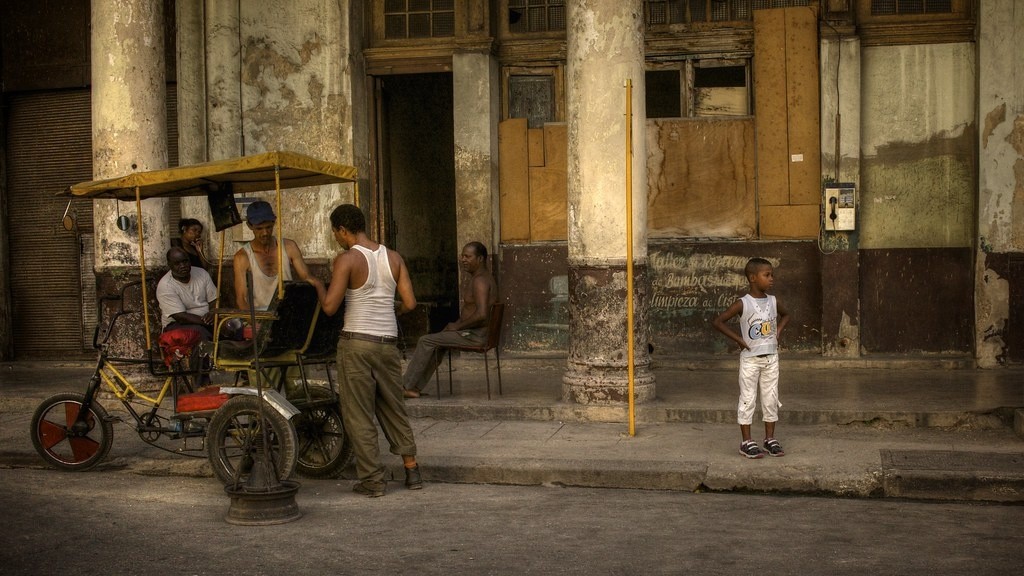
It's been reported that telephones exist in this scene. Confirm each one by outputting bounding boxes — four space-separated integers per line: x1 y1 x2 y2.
825 183 856 231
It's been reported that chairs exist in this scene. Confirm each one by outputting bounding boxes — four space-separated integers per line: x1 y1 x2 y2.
435 302 506 400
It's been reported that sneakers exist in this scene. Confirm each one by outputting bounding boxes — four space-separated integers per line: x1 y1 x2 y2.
404 463 423 489
762 437 784 457
353 479 386 497
739 439 764 458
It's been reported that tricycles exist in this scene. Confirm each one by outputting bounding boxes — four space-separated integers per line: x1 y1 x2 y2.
27 148 372 489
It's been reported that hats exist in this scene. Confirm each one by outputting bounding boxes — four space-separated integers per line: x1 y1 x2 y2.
247 201 275 224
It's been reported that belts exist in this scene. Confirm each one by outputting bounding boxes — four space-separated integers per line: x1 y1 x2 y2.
341 331 396 344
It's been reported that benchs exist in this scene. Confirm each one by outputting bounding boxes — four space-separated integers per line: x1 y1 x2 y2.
213 281 345 369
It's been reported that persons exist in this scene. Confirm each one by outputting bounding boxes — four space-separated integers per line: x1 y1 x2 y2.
233 200 312 421
170 217 208 268
305 203 424 497
156 246 220 387
713 257 791 458
402 241 499 399
